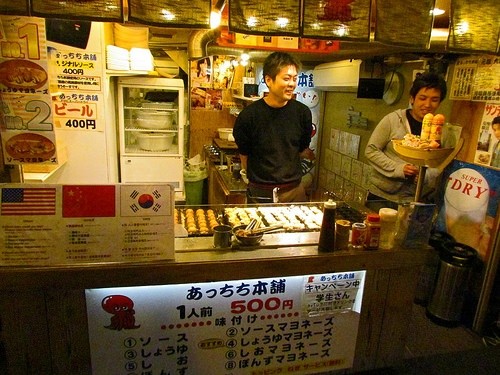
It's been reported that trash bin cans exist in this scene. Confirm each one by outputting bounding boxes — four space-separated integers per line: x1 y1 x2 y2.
414 231 485 329
184 163 208 205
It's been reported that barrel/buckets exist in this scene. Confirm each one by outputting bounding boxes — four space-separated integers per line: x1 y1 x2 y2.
413 230 455 305
425 242 478 327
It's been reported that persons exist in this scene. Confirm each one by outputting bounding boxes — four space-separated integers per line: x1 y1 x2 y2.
194 56 234 89
232 51 313 204
364 73 446 213
489 117 500 168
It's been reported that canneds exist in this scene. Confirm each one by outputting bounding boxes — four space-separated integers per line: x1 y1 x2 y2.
351 215 381 250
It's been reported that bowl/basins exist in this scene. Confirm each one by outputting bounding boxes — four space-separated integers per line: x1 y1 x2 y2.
232 225 264 245
106 24 154 71
240 169 249 185
138 112 173 129
137 133 176 151
217 127 233 140
390 137 454 159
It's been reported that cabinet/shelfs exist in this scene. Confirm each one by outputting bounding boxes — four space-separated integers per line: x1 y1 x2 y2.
226 95 252 117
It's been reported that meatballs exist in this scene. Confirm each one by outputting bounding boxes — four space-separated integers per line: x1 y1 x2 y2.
173 209 220 234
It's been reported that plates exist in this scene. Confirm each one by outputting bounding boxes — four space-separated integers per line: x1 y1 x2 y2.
0 59 48 90
4 133 55 163
475 153 491 164
142 101 174 109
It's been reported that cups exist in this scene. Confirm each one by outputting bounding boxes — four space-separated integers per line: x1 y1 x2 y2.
443 188 490 249
394 193 437 249
213 225 234 248
232 163 240 180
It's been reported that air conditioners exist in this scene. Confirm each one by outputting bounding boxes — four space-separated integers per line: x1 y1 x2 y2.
313 59 364 93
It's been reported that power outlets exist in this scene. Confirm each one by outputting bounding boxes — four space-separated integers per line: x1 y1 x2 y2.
413 70 425 82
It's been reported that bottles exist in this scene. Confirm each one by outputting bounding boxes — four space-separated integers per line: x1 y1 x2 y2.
378 207 398 250
363 213 382 250
429 114 444 141
317 199 337 252
335 219 351 249
351 222 366 248
421 113 434 139
